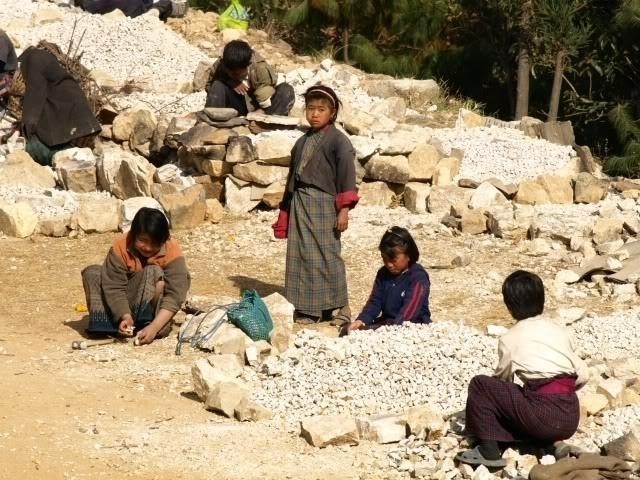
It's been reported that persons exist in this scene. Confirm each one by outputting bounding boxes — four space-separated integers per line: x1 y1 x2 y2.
80 207 191 346
456 270 579 466
204 39 295 116
347 226 432 337
0 27 19 120
273 86 360 326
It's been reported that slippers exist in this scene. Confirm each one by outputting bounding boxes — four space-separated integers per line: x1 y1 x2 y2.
555 445 571 459
455 444 508 468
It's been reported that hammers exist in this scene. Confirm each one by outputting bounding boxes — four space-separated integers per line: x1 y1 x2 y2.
72 338 114 350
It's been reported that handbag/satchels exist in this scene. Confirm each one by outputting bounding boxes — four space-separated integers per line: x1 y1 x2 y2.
224 288 274 341
216 0 250 33
